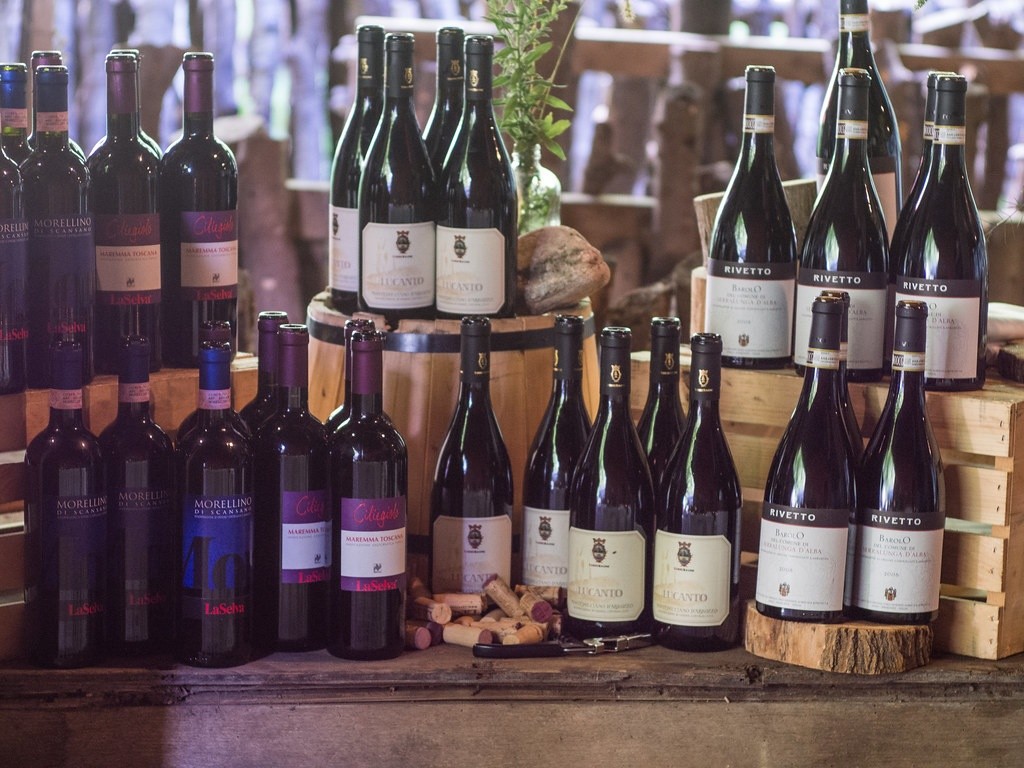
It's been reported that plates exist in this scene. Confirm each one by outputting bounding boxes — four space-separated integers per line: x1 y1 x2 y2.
884 72 988 392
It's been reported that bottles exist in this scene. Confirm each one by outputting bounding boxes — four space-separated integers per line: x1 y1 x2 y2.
522 314 592 640
329 25 439 319
177 322 255 668
24 343 111 669
854 300 946 626
816 1 902 249
87 49 162 375
239 312 329 653
99 334 176 667
706 65 797 369
422 26 516 320
323 319 407 660
162 52 238 366
567 326 655 642
791 69 889 383
756 289 865 622
429 315 514 621
0 51 94 394
636 316 741 649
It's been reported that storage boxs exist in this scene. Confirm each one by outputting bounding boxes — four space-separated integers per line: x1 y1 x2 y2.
0 350 256 667
631 343 1024 662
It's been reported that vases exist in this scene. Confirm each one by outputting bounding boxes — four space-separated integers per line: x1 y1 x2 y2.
510 140 558 240
